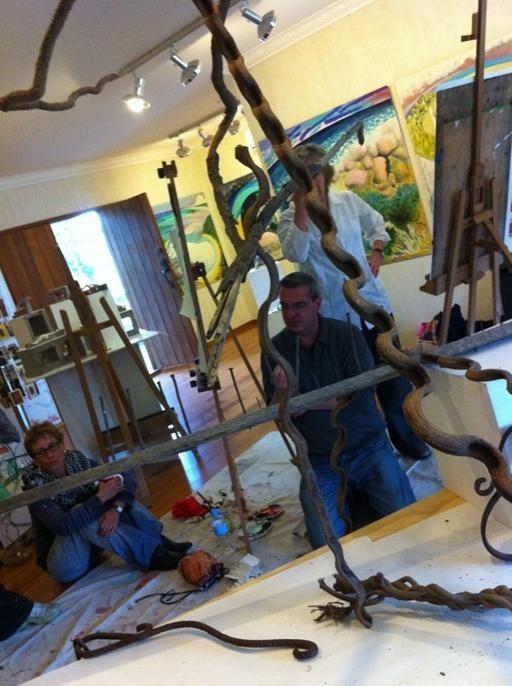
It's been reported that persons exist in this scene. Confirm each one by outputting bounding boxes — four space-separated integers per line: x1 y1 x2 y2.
21 420 192 584
261 272 417 550
277 143 431 460
0 582 60 642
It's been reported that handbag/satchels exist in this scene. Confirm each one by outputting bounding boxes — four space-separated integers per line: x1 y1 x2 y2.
437 304 466 342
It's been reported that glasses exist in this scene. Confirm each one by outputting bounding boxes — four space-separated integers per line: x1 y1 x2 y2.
276 298 313 311
33 440 59 456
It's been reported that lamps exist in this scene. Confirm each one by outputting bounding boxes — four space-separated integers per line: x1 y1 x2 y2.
237 1 276 42
120 70 151 117
165 43 202 88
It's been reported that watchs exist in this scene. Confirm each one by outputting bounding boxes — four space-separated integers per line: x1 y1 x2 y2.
111 504 124 513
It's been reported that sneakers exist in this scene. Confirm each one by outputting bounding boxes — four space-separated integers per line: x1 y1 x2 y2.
153 535 192 571
27 602 60 623
405 444 432 459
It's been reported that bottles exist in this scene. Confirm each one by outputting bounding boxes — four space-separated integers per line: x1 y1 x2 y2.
210 509 236 550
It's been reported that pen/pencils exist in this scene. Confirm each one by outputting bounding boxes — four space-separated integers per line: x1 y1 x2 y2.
197 491 214 508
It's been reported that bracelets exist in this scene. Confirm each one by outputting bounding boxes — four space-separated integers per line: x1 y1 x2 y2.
374 248 383 256
